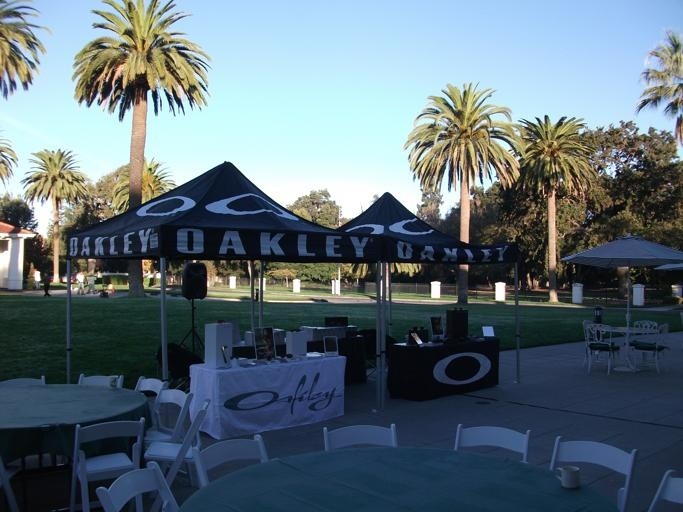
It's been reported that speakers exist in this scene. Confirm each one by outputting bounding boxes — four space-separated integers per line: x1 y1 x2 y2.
157 343 202 377
446 310 468 338
182 263 207 299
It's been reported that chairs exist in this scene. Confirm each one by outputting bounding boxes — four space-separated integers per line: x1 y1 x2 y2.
97 461 180 512
76 373 123 388
143 381 195 486
358 331 397 378
65 417 146 512
454 425 532 465
192 434 267 489
137 375 170 415
144 399 211 512
582 319 669 376
0 376 46 384
550 437 637 512
323 423 398 450
0 451 22 512
648 470 683 512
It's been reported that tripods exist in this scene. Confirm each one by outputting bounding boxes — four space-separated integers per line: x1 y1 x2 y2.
181 299 204 354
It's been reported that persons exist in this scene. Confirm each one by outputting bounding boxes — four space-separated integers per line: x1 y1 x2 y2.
44 268 52 296
558 235 682 372
76 271 97 295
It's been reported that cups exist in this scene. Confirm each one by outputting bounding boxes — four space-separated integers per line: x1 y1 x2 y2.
554 465 581 488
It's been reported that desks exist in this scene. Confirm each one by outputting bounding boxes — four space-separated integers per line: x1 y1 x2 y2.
1 383 152 512
179 446 620 512
396 338 500 401
189 353 346 440
235 336 366 384
